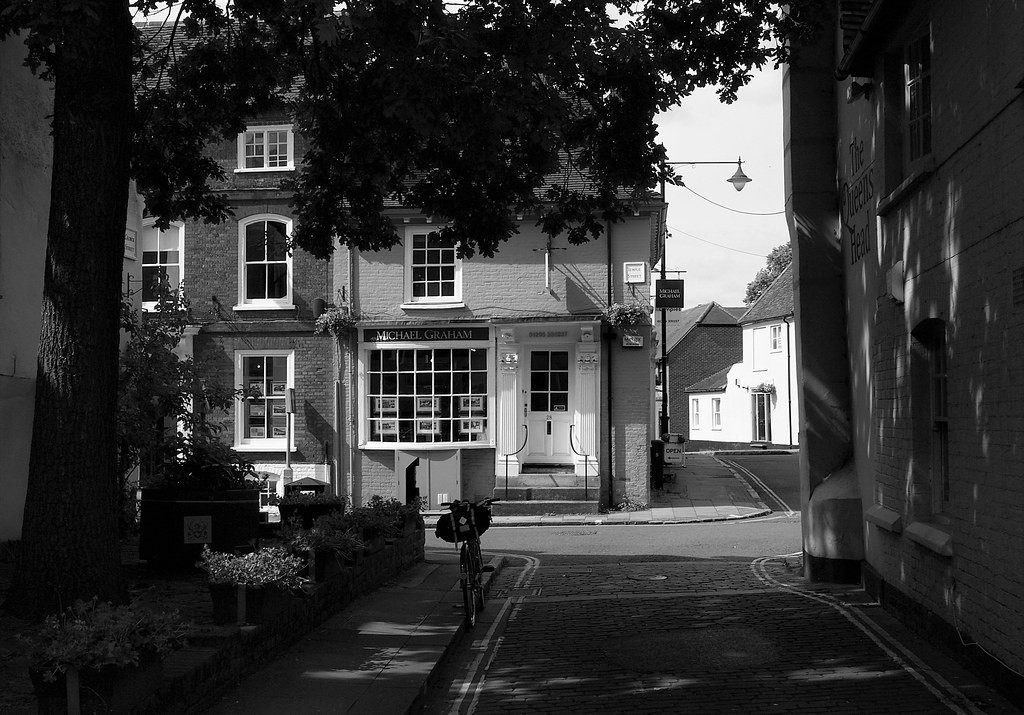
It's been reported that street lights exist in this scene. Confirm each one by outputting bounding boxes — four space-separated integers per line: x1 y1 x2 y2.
660 156 753 467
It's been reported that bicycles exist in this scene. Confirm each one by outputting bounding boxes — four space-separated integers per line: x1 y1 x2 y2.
439 496 503 630
